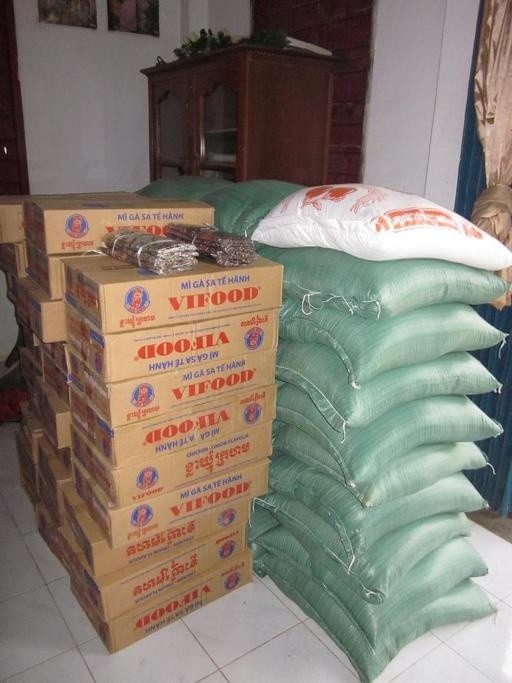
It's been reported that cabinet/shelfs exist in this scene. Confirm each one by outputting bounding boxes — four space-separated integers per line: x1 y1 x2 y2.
136 40 336 185
